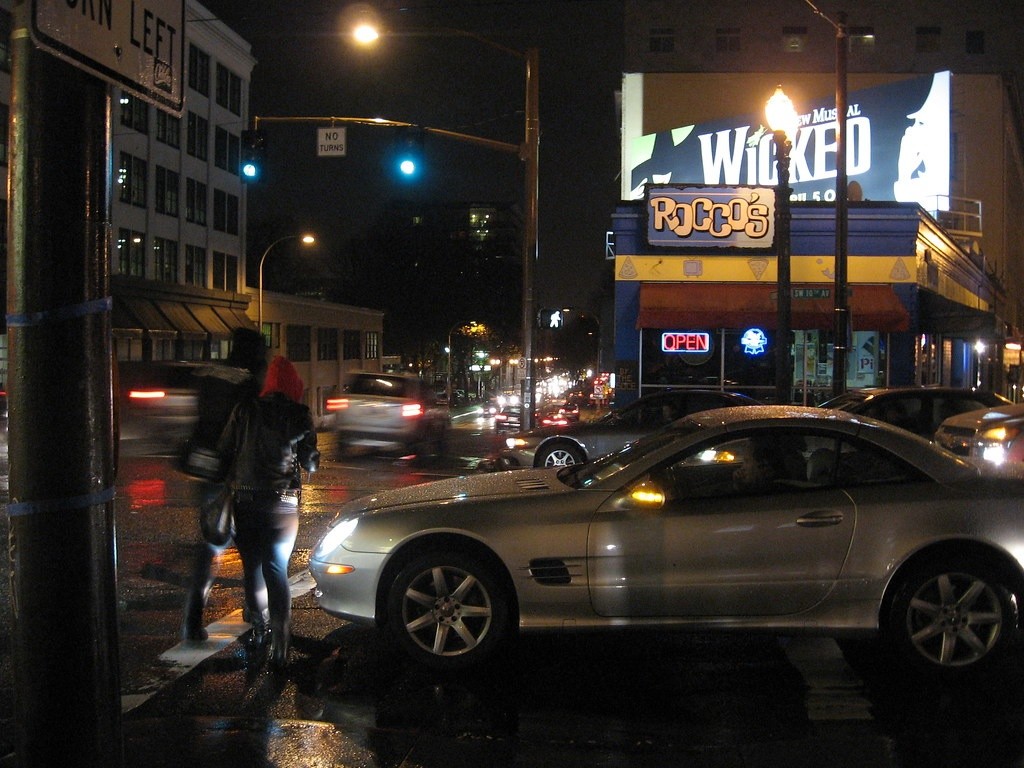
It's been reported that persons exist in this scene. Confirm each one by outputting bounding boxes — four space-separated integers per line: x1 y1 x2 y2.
184 327 269 647
659 400 679 423
216 356 320 672
878 402 905 429
730 435 787 497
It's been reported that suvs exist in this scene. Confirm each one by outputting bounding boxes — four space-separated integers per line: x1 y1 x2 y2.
935 403 1024 480
325 370 450 465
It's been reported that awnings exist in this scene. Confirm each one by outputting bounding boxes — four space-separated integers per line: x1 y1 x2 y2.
110 294 260 342
634 282 909 334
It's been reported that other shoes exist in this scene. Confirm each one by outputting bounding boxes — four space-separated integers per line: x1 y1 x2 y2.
252 624 271 644
267 643 287 662
184 626 209 642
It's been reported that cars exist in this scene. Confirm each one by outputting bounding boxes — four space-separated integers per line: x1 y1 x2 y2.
309 407 1024 691
499 389 764 471
819 385 1014 441
478 379 597 433
125 359 202 423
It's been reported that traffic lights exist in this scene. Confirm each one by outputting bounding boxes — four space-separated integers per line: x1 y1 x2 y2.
539 307 563 329
240 130 265 183
394 126 425 186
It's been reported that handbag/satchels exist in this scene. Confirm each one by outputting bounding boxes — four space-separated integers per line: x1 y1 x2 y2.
169 438 231 483
198 483 234 548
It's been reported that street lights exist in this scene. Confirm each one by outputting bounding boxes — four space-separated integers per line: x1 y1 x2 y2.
448 321 478 406
354 22 540 433
764 83 798 405
259 234 315 338
490 359 501 390
509 359 518 389
834 25 876 398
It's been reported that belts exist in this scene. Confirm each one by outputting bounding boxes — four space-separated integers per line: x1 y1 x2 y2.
239 493 298 505
